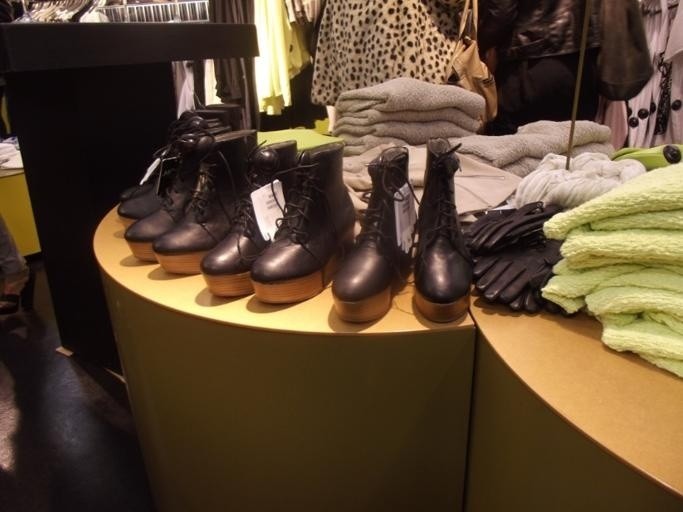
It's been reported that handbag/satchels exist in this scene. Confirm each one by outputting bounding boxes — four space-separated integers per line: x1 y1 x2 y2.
440 0 499 123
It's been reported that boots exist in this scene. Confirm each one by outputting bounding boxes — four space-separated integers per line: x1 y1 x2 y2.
248 136 358 309
328 141 417 326
121 124 237 265
197 135 307 301
413 135 474 327
152 129 269 279
116 102 244 233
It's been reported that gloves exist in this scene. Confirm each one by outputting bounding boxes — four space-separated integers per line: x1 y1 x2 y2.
465 199 564 316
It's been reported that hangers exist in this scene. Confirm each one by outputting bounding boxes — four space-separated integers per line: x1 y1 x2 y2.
12 0 113 24
